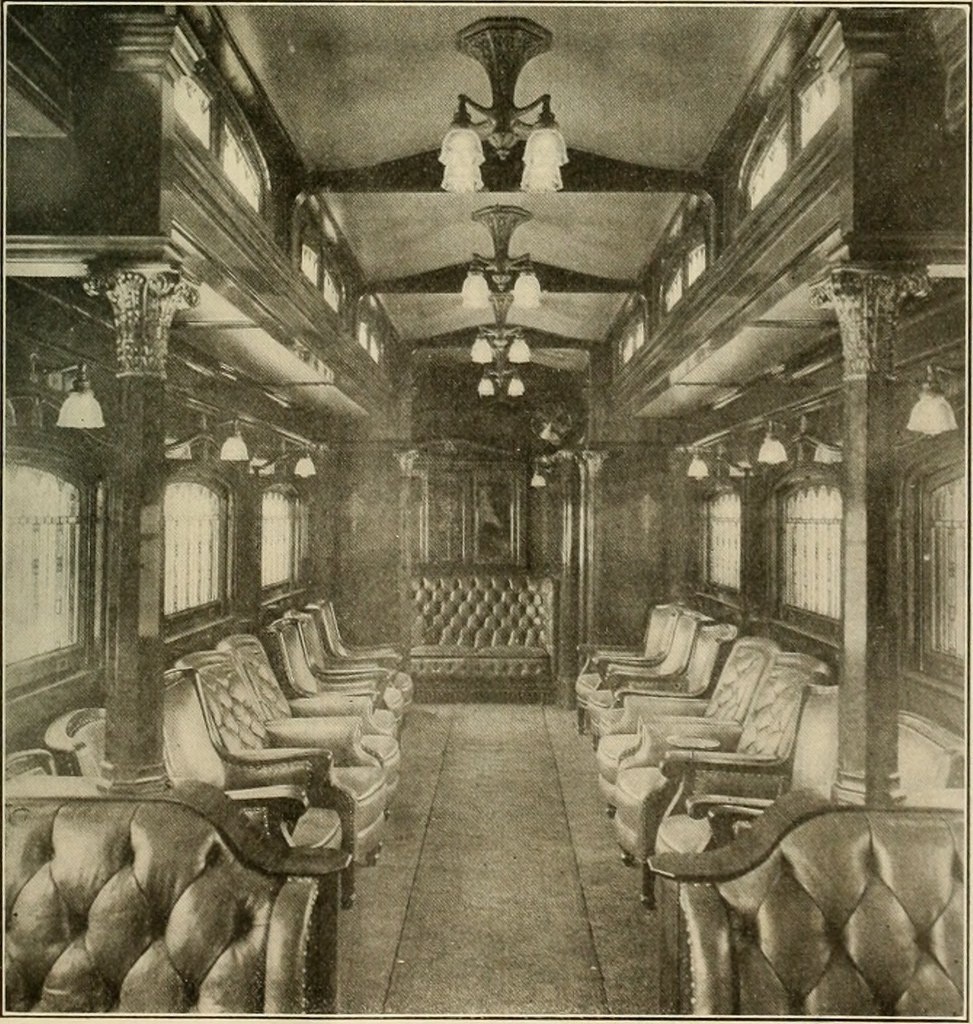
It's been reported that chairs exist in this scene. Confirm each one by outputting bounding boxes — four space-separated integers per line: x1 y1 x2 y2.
307 596 415 708
283 599 405 731
178 648 386 851
220 632 401 807
899 711 966 792
647 789 967 1021
41 705 355 910
641 684 839 908
596 623 742 754
157 667 387 894
590 605 715 751
257 604 398 741
597 633 786 818
2 775 350 1015
576 598 687 735
612 651 836 864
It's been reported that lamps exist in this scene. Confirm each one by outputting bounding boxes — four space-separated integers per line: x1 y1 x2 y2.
469 296 532 363
199 417 249 462
540 418 564 443
686 447 749 482
763 416 842 466
906 364 970 437
28 352 107 428
439 14 570 194
530 462 547 490
460 204 544 311
255 440 320 479
477 350 526 397
